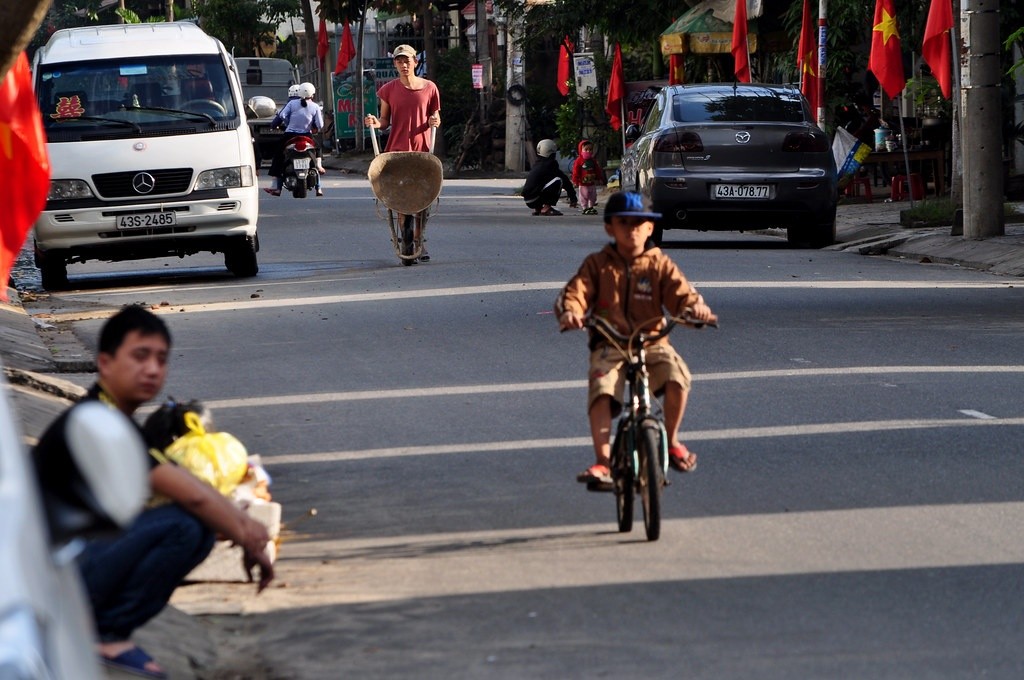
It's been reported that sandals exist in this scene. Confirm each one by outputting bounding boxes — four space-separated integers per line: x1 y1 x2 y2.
577 463 614 484
669 442 698 473
582 209 594 215
590 207 598 215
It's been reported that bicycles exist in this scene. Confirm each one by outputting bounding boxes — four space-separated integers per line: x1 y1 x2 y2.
558 307 721 540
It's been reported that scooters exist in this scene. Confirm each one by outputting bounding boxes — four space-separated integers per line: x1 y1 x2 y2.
272 126 319 199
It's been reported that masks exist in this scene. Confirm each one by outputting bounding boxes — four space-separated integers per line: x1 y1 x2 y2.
581 152 592 159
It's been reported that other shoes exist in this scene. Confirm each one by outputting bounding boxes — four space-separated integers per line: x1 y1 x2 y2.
319 167 326 174
271 191 281 196
265 188 278 193
420 253 430 262
316 190 324 196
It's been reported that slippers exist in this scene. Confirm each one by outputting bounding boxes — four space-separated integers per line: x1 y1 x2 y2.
540 207 563 216
98 645 166 680
532 207 542 215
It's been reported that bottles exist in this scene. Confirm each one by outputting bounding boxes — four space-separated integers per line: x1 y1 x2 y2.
884 135 894 152
132 94 139 107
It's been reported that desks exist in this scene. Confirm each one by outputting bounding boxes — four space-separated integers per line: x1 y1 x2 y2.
862 149 945 197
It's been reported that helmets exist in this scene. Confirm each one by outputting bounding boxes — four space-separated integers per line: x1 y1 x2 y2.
299 82 316 97
288 84 300 98
537 139 557 157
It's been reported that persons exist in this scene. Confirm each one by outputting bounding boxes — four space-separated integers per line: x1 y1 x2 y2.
522 139 578 216
264 82 325 197
554 189 711 484
572 140 604 215
30 303 275 680
365 44 441 264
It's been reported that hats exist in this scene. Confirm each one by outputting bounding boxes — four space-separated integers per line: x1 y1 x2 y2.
604 190 662 217
393 44 417 59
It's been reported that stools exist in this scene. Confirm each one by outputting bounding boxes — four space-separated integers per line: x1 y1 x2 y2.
845 178 872 200
891 173 922 201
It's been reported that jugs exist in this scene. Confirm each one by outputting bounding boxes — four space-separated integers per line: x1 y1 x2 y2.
873 127 891 152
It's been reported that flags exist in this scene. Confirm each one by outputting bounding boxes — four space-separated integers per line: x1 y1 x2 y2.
669 17 684 86
0 49 51 301
334 18 356 75
317 18 329 72
606 45 625 131
557 34 574 96
867 0 905 100
922 0 955 100
731 0 750 82
797 0 818 123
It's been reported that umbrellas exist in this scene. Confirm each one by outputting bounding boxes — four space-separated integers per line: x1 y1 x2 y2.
661 0 792 56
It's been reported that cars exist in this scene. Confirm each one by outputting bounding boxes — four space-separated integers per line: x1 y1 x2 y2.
618 81 842 246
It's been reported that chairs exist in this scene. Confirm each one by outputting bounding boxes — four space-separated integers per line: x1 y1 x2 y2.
180 79 214 107
54 91 88 116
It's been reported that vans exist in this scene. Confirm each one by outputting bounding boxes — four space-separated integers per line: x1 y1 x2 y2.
24 21 280 287
232 55 299 173
